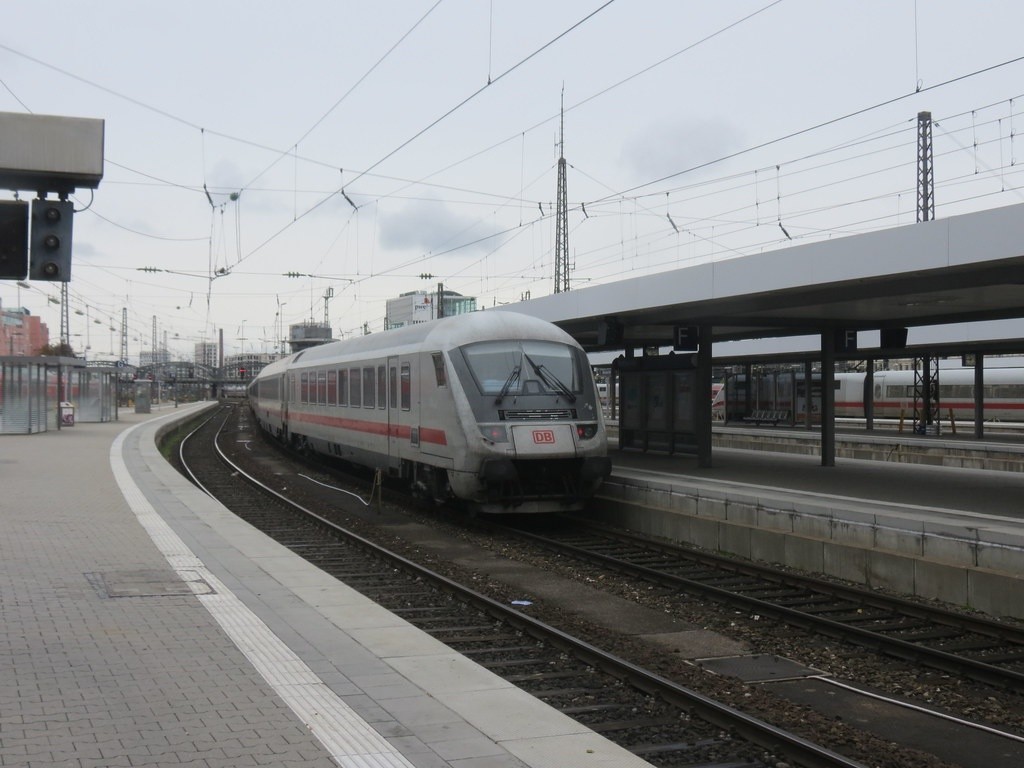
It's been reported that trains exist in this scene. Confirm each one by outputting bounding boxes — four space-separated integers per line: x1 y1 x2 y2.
597 364 1024 427
248 309 612 519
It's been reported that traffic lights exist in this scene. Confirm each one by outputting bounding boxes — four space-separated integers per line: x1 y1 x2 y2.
30 198 73 280
0 198 28 281
